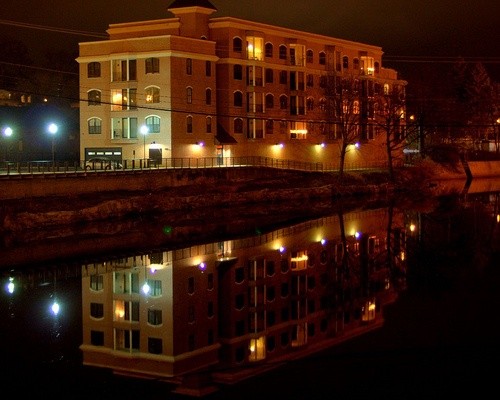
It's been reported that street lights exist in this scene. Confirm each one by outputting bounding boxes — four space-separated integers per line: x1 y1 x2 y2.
140 124 149 168
48 121 57 172
4 124 12 168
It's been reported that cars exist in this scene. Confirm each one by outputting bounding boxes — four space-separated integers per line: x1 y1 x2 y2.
81 157 123 170
